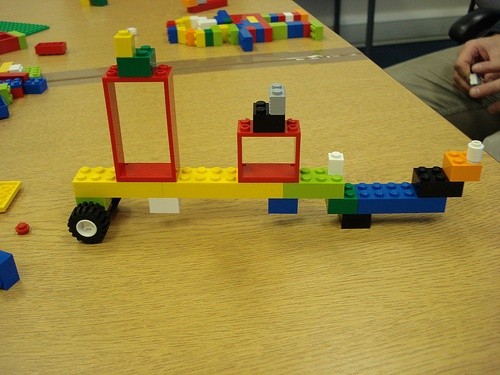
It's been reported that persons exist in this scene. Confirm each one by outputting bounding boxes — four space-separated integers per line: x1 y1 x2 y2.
381 20 500 163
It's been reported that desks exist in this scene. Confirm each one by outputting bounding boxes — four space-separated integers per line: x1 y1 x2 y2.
0 0 500 375
